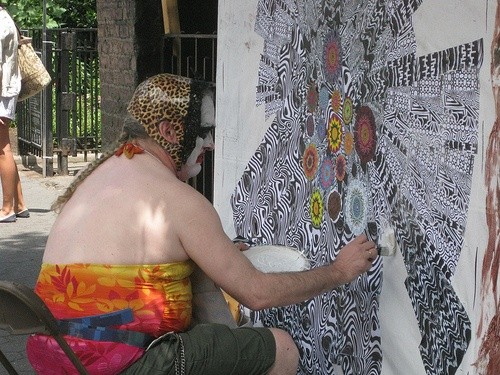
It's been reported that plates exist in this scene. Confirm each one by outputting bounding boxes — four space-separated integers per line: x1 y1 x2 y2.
241 245 307 274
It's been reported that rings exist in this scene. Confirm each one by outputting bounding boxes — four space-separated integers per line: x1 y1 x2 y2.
368 250 372 258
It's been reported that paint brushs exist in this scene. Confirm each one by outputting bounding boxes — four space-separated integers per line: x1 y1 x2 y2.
338 243 395 257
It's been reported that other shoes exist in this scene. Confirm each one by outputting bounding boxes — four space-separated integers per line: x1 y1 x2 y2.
0 214 17 223
15 209 31 218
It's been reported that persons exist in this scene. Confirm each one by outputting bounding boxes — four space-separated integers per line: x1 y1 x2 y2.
25 73 379 375
0 0 29 223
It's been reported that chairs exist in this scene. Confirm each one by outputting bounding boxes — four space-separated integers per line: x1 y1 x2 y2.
0 281 91 375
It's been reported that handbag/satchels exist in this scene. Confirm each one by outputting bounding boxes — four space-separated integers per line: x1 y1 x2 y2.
16 32 52 103
